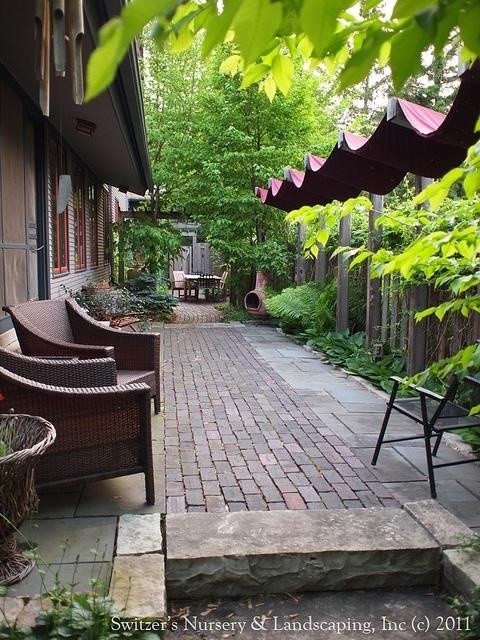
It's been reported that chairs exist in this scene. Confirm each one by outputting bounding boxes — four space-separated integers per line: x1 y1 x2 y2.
171 271 229 303
369 370 480 498
0 294 161 506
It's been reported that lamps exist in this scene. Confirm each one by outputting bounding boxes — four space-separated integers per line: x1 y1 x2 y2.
73 117 97 155
